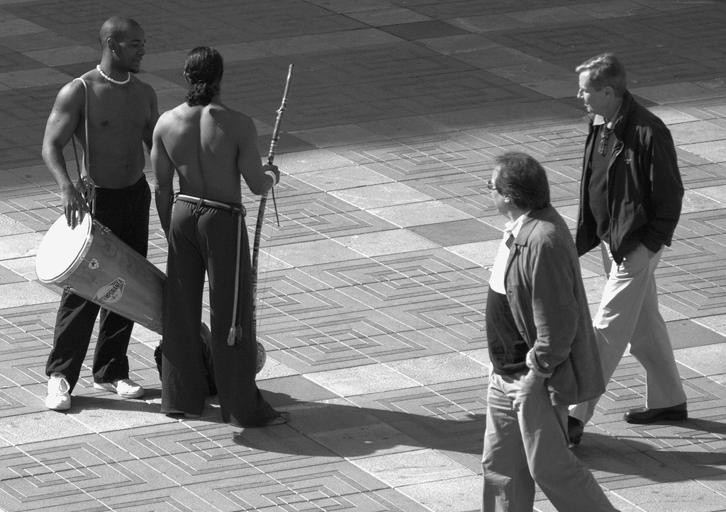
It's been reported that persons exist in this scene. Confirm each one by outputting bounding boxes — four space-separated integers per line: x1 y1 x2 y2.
480 151 621 512
568 50 688 446
149 45 290 426
40 15 160 411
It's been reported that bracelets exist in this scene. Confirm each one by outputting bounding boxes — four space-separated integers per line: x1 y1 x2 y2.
265 171 279 186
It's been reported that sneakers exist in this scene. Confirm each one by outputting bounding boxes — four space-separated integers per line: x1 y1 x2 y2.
230 411 290 427
93 378 144 398
45 372 71 411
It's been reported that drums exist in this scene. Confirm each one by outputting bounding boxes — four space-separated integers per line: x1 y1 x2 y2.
255 342 265 374
35 209 167 336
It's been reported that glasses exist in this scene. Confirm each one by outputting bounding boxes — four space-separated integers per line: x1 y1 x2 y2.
485 180 497 191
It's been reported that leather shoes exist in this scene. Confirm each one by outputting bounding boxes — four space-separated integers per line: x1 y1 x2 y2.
624 403 687 424
569 414 584 446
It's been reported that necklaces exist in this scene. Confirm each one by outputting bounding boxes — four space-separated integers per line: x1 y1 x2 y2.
97 63 133 86
